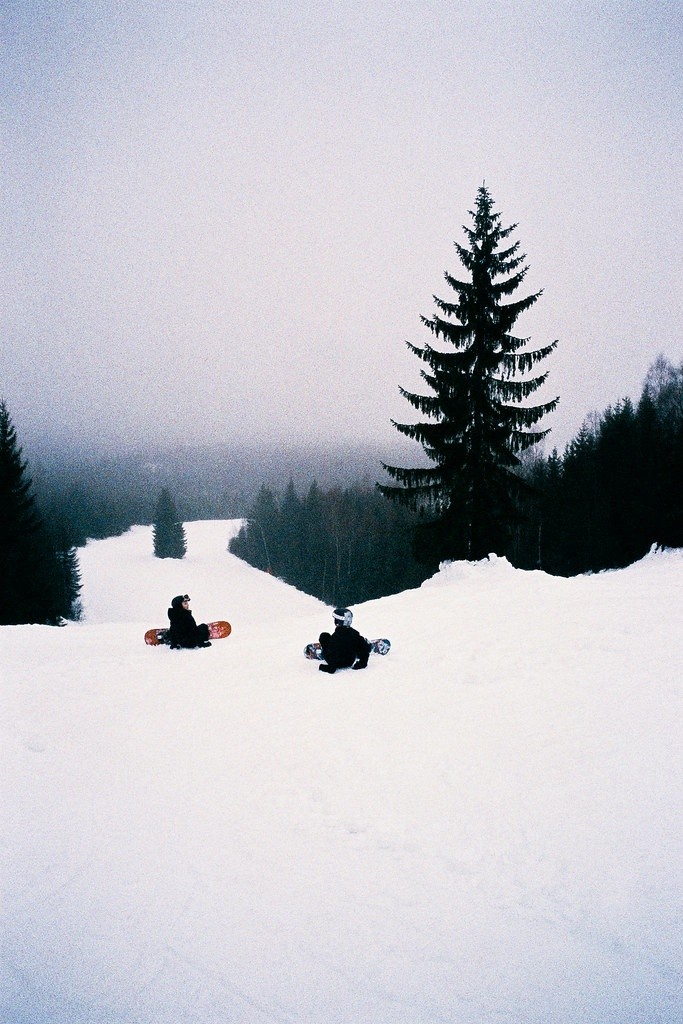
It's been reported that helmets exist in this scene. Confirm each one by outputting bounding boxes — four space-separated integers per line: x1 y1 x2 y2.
333 608 354 625
172 594 190 608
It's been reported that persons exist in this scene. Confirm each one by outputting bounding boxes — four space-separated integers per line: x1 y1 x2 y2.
319 609 369 674
168 595 211 650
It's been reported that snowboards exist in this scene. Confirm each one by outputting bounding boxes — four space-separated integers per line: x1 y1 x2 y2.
303 639 390 660
144 621 232 646
